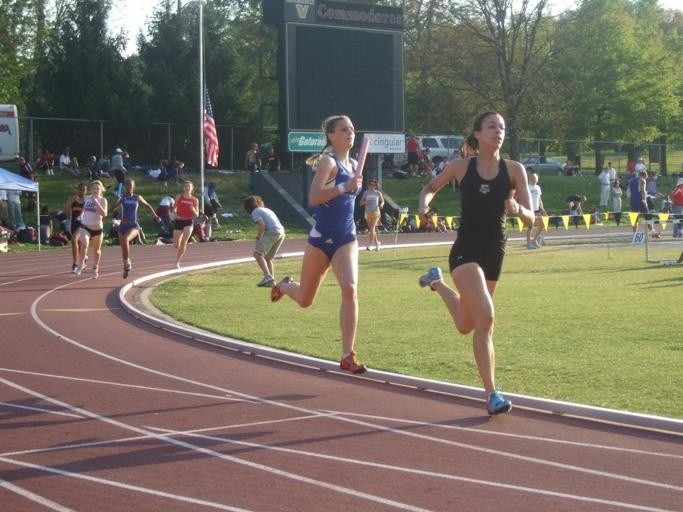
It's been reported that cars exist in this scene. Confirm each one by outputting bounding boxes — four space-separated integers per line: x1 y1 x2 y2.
522 157 567 176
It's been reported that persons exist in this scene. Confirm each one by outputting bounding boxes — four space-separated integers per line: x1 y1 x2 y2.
18 158 38 211
149 162 182 188
37 150 56 176
112 148 128 197
203 182 223 232
111 210 148 245
60 147 83 180
271 114 373 373
360 179 386 252
606 162 620 194
406 135 419 176
670 177 683 239
245 144 263 173
265 146 280 173
436 154 450 175
611 180 624 226
563 160 583 177
627 172 659 238
87 156 103 181
527 172 549 248
598 166 612 209
645 173 665 214
566 193 587 215
169 155 186 177
170 181 200 269
420 145 434 175
74 180 109 279
155 196 177 226
419 113 536 414
39 206 54 240
635 157 649 179
245 195 286 286
64 183 89 273
109 179 162 280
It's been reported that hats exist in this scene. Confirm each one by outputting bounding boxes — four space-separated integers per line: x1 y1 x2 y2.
114 147 124 153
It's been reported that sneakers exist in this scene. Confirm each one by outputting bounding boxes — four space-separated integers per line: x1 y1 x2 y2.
256 275 275 288
70 264 78 273
418 267 443 292
264 280 277 288
270 276 295 303
122 266 129 279
92 266 99 280
375 242 381 252
366 245 373 251
125 259 131 272
339 351 367 375
486 391 514 416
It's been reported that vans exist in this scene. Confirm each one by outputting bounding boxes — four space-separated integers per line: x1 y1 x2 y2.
405 136 463 165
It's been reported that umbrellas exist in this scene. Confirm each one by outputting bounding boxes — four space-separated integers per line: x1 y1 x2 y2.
0 167 37 194
